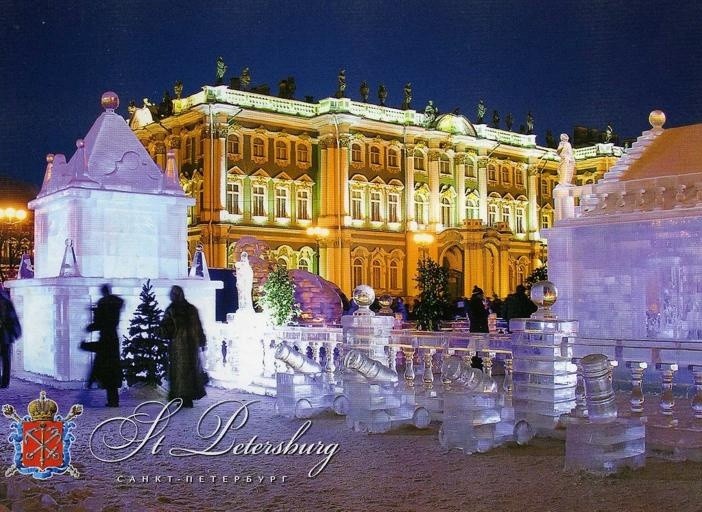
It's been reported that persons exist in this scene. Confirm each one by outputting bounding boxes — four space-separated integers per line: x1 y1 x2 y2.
80 283 124 407
150 286 209 408
0 295 21 388
125 56 536 138
468 283 538 332
556 132 574 189
